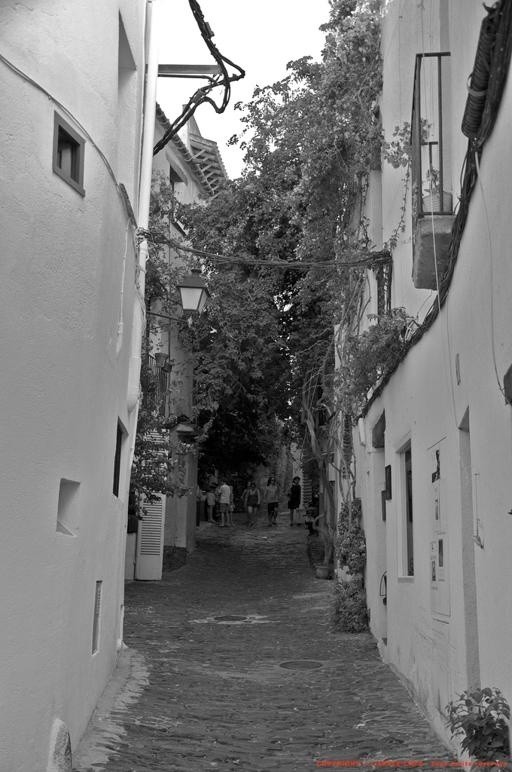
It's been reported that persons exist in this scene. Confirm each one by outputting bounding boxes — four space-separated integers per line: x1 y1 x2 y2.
196 476 302 526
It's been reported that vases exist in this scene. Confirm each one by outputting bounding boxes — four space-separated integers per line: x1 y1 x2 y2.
314 565 331 580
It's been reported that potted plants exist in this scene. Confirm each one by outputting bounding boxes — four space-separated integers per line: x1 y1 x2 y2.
418 170 454 215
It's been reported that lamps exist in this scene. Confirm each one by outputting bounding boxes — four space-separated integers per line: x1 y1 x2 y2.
144 258 212 337
